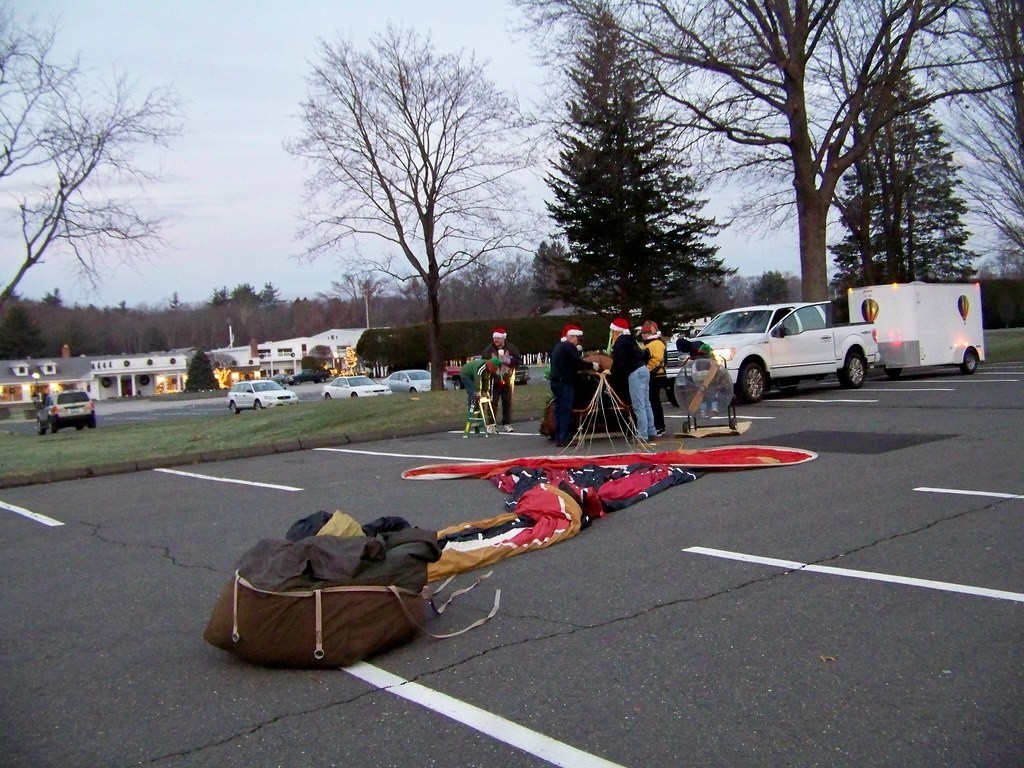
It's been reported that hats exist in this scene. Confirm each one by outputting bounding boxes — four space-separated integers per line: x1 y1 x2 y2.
560 324 584 352
643 320 659 332
486 358 501 373
610 318 631 335
492 327 506 339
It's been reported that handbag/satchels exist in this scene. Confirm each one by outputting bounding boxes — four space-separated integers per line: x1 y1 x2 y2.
203 528 442 666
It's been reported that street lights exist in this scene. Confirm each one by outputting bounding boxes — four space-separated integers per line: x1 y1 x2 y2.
32 371 40 411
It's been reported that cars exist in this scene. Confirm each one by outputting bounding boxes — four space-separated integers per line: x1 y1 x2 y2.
321 374 392 400
270 374 294 385
381 369 449 392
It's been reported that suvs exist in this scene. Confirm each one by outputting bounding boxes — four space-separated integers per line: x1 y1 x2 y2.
37 388 96 435
227 380 298 415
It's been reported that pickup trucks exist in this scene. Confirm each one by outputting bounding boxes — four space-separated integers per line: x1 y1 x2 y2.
664 301 881 407
446 356 530 390
291 369 330 386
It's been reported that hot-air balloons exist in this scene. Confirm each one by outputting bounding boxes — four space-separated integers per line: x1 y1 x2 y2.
862 299 878 323
958 295 969 325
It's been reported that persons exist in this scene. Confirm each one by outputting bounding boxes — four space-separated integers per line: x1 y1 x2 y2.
640 322 667 438
601 317 658 442
549 324 599 447
676 337 719 417
460 358 500 414
482 328 522 437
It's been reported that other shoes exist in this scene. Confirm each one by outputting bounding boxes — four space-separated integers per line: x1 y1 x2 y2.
648 435 656 441
487 426 497 435
556 438 577 446
710 411 719 416
468 403 480 415
629 437 649 444
656 428 666 439
502 423 514 432
700 410 709 418
